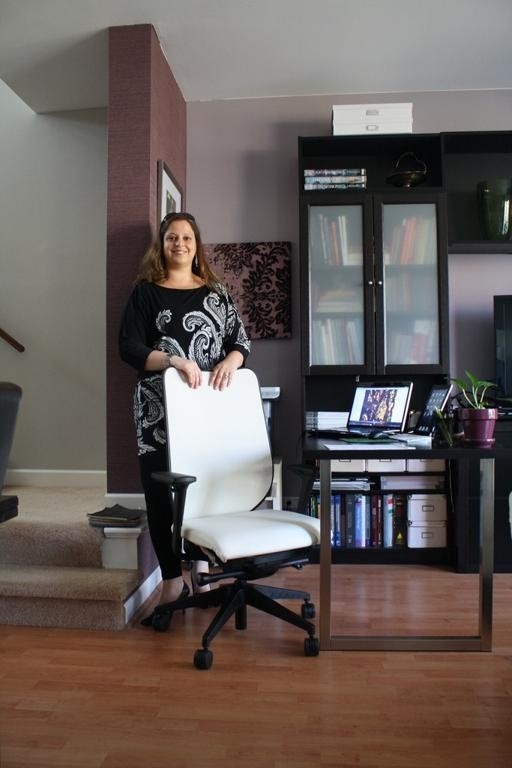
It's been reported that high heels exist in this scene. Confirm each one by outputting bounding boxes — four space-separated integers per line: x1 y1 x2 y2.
142 570 212 628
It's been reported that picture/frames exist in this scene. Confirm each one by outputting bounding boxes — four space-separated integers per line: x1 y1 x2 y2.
157 158 185 233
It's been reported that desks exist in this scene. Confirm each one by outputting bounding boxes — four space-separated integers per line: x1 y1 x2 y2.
303 430 503 655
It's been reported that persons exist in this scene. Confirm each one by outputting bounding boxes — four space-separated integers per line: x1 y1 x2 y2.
115 210 252 632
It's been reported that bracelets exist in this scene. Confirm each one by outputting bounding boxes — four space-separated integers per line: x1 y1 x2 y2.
161 352 177 370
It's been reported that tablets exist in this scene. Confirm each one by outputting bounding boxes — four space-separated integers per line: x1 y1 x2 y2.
413 383 453 438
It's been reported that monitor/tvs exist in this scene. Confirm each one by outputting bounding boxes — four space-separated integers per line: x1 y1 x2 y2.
492 293 512 421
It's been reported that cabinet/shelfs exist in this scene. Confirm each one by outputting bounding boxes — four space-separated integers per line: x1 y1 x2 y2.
440 129 512 254
451 419 512 574
291 129 455 565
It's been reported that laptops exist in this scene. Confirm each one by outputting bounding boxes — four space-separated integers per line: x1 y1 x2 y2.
305 376 413 439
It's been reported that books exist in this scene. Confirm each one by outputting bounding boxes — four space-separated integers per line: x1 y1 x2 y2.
301 168 440 365
302 408 405 550
84 503 148 540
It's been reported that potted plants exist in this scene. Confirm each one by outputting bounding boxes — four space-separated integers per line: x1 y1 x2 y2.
444 369 499 449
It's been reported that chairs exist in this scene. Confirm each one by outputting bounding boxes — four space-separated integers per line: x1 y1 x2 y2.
150 366 334 670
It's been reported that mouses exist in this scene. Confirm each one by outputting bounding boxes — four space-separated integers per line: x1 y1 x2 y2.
368 430 389 439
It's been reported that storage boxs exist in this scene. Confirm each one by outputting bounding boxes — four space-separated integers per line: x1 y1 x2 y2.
332 102 414 136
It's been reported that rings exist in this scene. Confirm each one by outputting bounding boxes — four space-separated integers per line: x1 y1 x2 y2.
222 374 229 379
